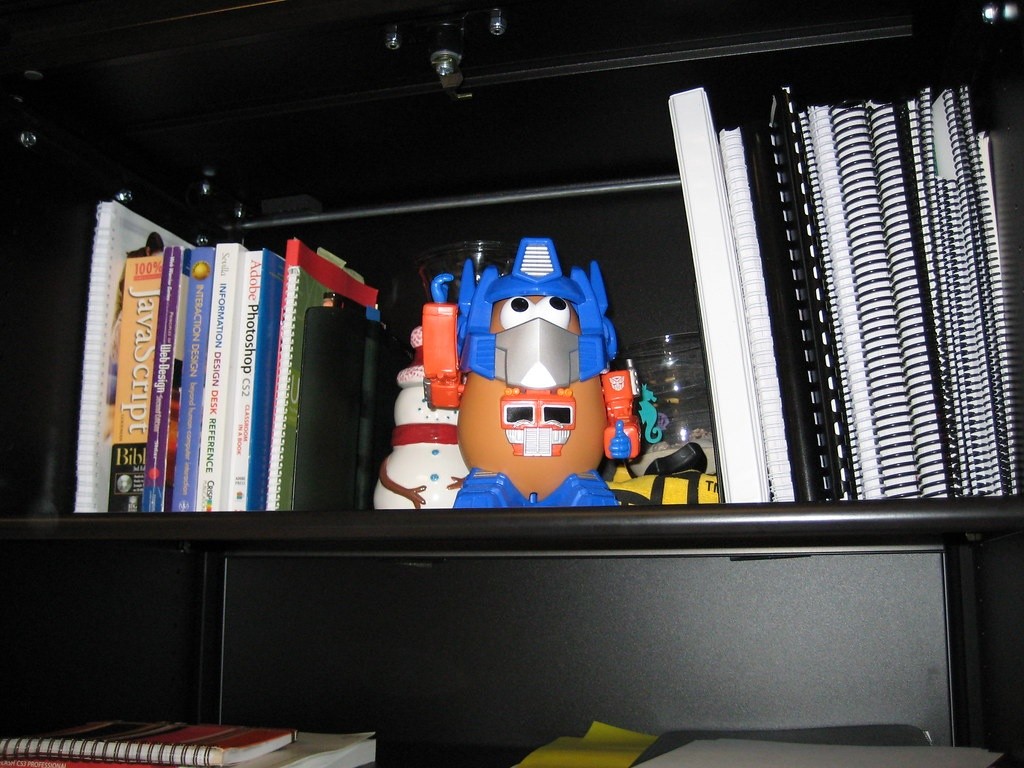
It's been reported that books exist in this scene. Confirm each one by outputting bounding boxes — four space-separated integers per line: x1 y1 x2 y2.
0 720 378 768
76 201 413 513
667 85 1021 503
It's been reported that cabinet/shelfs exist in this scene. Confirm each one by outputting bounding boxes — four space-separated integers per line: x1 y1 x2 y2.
0 0 1024 728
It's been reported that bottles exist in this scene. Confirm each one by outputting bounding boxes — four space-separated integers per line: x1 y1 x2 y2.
414 240 519 306
626 331 717 478
